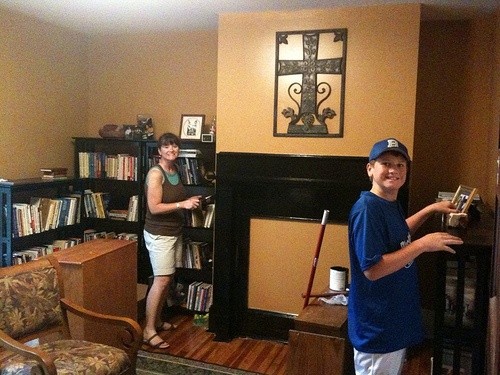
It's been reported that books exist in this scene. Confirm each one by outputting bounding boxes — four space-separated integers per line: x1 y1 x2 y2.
437 192 480 375
2 152 215 312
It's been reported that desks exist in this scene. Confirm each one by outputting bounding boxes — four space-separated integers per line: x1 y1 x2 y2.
285 285 351 374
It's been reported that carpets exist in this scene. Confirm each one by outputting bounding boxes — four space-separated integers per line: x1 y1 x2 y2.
136 349 266 375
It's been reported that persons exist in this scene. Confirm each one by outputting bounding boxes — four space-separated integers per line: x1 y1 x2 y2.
348 139 463 375
143 132 200 349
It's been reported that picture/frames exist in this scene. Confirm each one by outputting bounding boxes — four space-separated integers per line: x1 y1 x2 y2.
451 184 480 212
177 114 205 140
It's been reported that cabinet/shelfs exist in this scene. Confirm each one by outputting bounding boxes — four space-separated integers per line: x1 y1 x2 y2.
0 135 215 354
432 242 495 375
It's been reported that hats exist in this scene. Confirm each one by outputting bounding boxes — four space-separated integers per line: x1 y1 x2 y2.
368 138 411 163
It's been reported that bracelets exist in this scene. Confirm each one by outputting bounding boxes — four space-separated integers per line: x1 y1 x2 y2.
177 202 179 211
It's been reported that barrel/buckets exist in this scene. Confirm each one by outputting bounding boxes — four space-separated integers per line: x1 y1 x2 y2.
329 267 348 292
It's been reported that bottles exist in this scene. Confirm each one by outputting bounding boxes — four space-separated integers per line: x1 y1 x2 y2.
209 115 216 142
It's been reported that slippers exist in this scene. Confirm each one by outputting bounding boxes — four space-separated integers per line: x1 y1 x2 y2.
143 333 169 349
154 320 179 331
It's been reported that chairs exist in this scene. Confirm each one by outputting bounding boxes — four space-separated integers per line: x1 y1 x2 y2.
0 255 143 375
